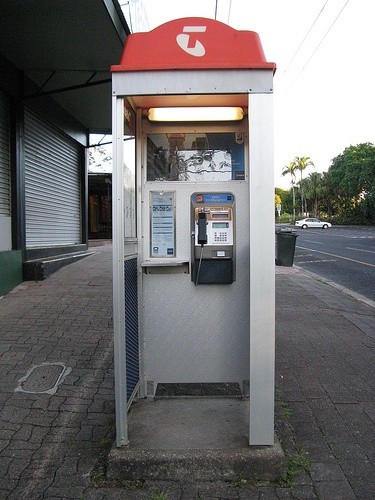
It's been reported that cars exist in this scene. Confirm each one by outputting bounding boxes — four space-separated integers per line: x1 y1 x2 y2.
295 218 332 229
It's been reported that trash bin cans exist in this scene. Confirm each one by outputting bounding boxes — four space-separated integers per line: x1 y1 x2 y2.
276 229 300 269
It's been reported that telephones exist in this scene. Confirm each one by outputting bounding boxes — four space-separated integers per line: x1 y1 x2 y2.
193 205 234 262
